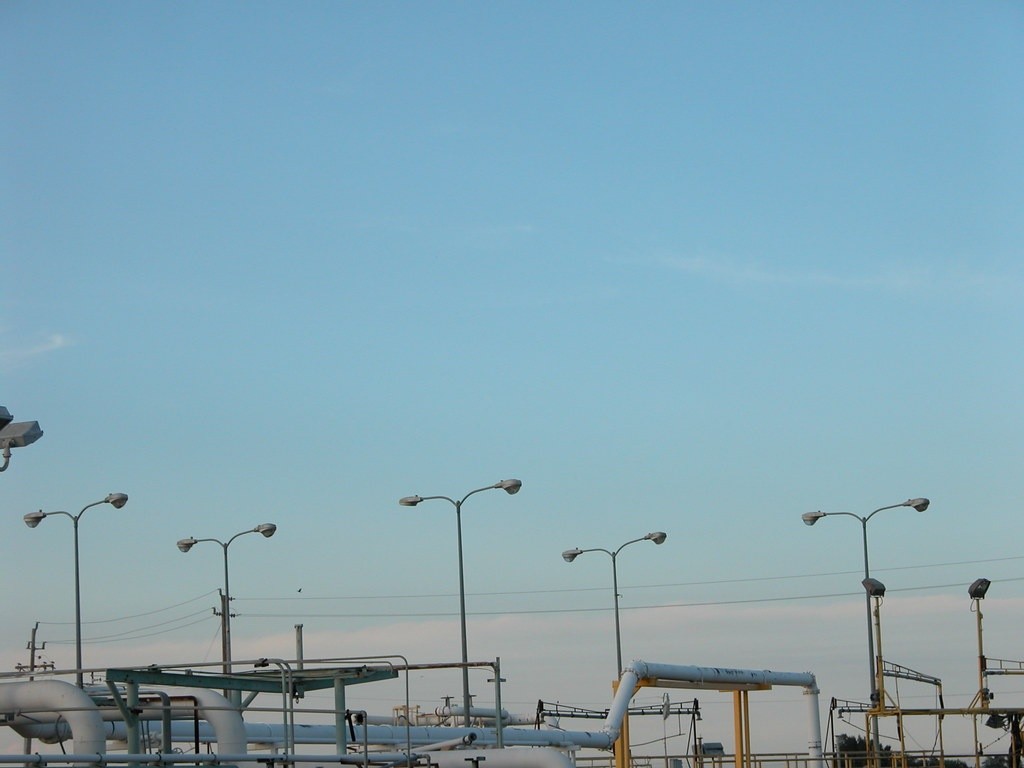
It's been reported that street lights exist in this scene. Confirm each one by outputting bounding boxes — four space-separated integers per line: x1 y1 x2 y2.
561 531 669 684
21 493 129 691
178 523 278 701
398 478 523 727
802 496 930 767
